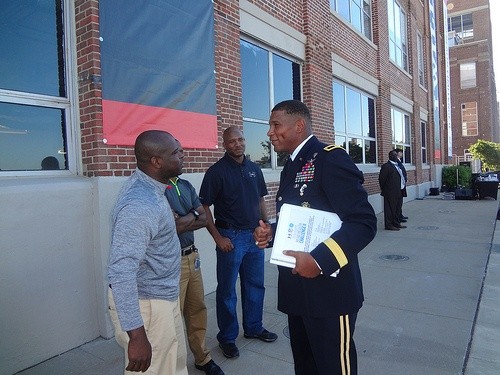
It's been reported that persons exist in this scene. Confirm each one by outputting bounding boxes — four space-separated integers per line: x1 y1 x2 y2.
165 139 224 375
108 130 188 375
253 99 378 375
379 148 409 231
199 126 278 358
41 156 59 170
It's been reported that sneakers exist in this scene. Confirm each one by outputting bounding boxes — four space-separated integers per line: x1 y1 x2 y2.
245 326 278 343
219 341 240 358
195 359 225 375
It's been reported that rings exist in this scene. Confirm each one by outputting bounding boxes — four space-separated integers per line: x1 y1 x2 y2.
176 216 177 218
255 241 259 245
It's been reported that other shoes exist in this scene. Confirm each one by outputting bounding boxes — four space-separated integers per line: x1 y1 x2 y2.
401 220 407 222
398 225 406 228
402 216 408 219
385 226 400 230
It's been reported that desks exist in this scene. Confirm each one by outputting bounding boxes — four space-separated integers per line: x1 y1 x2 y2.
477 178 499 200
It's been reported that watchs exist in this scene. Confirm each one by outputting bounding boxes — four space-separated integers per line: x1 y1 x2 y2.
189 209 199 220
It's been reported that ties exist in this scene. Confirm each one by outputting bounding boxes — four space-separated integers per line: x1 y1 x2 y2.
286 159 291 176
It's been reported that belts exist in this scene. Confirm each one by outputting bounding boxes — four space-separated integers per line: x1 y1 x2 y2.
109 284 111 288
182 245 195 256
